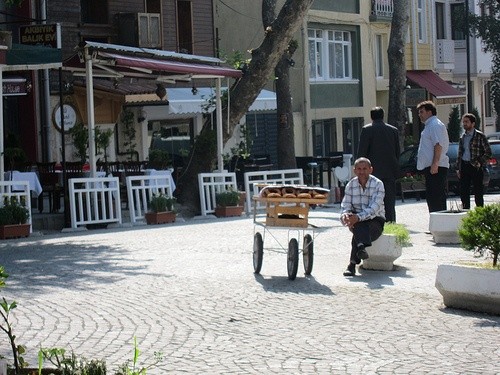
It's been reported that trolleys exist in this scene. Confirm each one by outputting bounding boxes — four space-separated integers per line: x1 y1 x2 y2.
250 183 330 280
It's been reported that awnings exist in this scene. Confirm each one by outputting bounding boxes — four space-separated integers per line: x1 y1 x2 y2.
405 69 466 98
162 88 278 115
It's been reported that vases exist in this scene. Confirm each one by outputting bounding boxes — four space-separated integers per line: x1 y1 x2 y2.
397 181 425 202
0 223 30 239
429 209 471 243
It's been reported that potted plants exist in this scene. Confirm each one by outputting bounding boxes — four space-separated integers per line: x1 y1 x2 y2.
435 201 500 315
363 221 412 270
215 191 243 217
144 196 177 224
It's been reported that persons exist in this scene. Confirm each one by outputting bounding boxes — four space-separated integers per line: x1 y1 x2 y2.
416 101 450 234
339 158 386 275
357 107 401 223
456 113 493 208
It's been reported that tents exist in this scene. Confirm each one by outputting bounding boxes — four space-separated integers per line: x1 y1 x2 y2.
0 44 69 206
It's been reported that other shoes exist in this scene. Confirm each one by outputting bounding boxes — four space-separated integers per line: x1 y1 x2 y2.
357 246 368 259
343 263 355 275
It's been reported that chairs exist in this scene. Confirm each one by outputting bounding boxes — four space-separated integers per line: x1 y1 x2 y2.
20 161 143 211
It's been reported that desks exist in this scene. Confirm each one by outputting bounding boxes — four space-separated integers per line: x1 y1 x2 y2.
50 170 114 213
111 169 177 210
4 172 43 215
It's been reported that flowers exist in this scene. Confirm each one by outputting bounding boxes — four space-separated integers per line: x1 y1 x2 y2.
396 174 425 182
0 194 29 225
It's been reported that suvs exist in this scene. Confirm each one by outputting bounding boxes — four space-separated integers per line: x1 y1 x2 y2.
486 139 500 187
392 140 491 195
